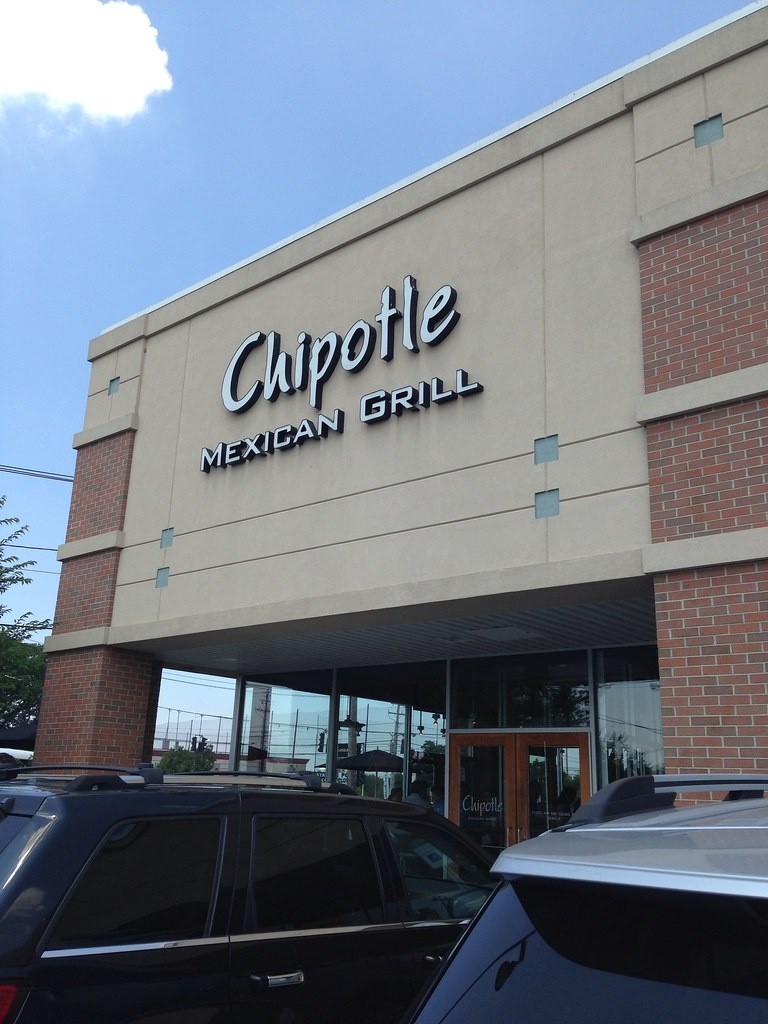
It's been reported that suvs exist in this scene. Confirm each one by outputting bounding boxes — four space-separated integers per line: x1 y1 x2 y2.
0 764 509 1024
407 773 768 1023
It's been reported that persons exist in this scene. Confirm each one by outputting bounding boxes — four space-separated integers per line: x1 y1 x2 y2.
387 780 445 817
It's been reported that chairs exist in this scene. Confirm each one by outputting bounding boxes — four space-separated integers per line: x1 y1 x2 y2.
323 839 381 920
256 841 322 925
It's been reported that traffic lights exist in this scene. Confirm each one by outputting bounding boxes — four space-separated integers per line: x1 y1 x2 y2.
190 736 196 751
318 732 324 752
202 737 208 749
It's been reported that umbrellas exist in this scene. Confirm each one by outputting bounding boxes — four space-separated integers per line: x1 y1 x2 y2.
312 747 427 798
